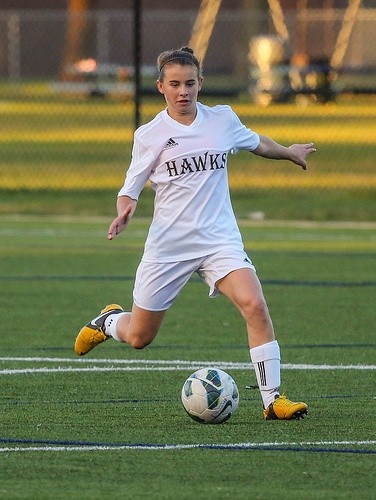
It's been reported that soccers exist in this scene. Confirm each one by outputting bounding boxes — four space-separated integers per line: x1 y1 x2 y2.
180 368 241 424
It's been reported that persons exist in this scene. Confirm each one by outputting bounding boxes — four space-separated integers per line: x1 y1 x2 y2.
73 46 318 420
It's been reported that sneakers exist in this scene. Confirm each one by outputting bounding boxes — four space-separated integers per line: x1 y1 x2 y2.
75 304 124 356
262 393 308 420
